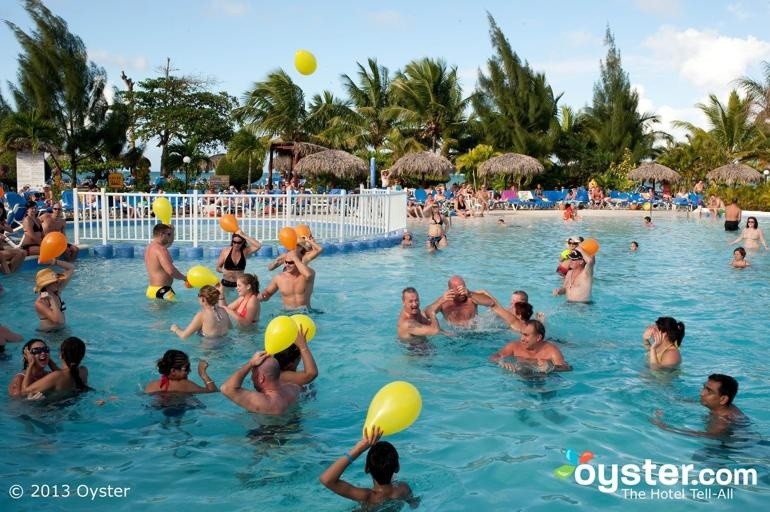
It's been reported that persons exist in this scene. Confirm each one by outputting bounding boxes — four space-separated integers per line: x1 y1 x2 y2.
1 180 770 512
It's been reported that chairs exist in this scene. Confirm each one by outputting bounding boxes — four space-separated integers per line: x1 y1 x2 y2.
0 188 197 230
197 187 410 214
411 186 704 210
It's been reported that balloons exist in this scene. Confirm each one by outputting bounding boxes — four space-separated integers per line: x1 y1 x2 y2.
295 50 317 75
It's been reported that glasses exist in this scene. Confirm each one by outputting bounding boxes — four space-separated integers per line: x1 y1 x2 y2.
54 208 61 211
233 241 242 245
284 260 295 264
31 346 50 355
568 242 578 246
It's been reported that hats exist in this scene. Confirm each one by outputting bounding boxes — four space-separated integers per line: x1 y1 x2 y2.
568 250 584 259
32 268 68 293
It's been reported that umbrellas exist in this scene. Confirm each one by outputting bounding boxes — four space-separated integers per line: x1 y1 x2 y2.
477 152 544 188
268 155 292 170
198 153 226 172
704 163 763 189
294 150 368 189
388 150 457 189
629 163 682 191
